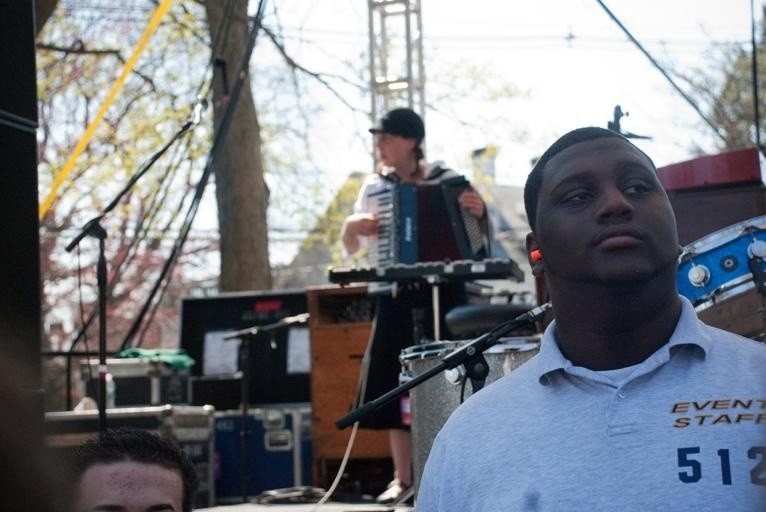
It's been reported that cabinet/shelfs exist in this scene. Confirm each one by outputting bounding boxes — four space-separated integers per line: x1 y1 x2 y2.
306 286 394 491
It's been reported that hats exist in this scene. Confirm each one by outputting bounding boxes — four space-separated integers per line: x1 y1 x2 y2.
369 107 426 138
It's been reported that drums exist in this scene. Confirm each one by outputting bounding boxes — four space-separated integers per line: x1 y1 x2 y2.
77 358 190 413
676 214 765 340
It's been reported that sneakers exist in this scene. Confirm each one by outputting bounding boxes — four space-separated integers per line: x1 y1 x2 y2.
375 476 413 506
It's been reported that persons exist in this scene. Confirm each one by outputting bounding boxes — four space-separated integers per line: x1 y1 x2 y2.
338 107 491 510
413 126 765 512
63 428 200 510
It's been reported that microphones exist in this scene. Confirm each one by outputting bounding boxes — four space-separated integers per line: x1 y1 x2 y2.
173 116 197 139
278 309 313 331
